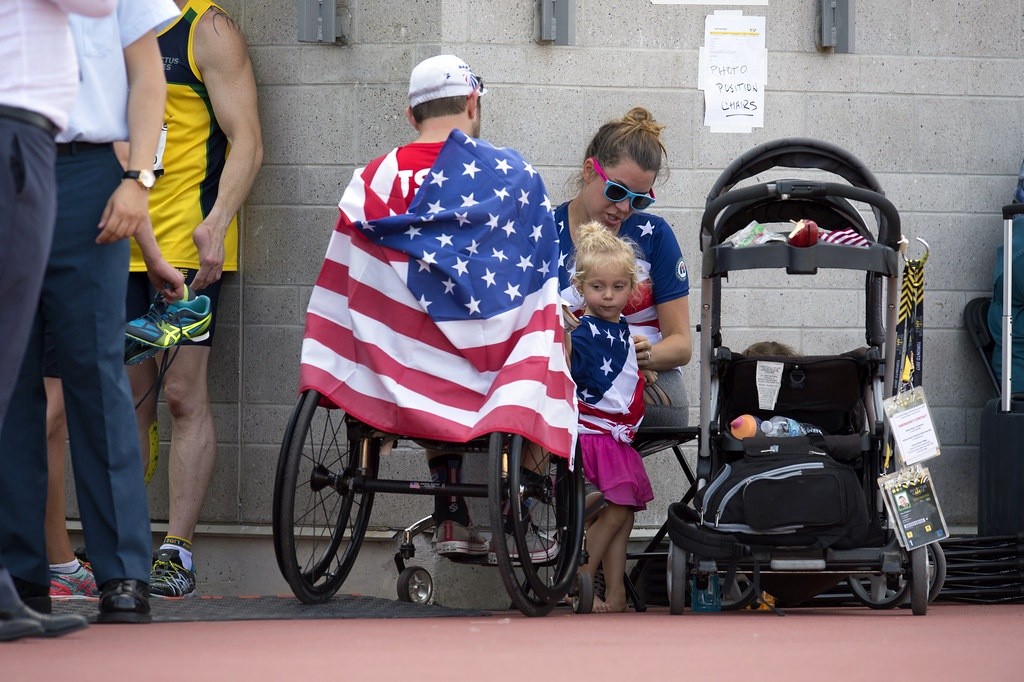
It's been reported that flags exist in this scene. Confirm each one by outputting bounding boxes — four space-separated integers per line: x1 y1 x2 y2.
300 131 580 470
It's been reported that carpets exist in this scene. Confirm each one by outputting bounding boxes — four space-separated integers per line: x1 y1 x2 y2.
51 594 491 623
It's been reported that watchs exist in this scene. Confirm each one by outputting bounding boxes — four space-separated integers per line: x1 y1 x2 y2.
121 169 156 190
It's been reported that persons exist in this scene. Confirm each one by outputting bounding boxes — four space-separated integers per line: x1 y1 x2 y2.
46 376 99 598
898 496 909 509
553 106 692 611
0 0 113 642
743 341 797 359
566 222 658 611
1 0 180 622
74 0 263 600
300 53 577 554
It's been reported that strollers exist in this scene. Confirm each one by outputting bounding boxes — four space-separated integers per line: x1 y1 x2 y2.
665 139 947 617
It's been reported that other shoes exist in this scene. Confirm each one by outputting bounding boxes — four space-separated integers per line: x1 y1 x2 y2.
584 481 609 522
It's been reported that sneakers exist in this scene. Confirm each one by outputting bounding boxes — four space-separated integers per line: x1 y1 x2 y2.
124 282 213 366
149 544 197 599
488 522 561 565
436 515 490 557
48 546 103 602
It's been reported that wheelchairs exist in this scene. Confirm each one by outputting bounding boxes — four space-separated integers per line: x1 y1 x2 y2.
273 375 596 618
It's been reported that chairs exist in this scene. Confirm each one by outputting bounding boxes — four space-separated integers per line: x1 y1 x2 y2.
509 425 762 613
964 297 1024 398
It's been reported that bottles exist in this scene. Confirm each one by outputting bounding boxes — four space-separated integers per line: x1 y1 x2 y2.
761 415 824 438
731 414 765 440
693 557 721 612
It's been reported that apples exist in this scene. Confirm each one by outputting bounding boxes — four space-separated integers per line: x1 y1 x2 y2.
787 219 818 247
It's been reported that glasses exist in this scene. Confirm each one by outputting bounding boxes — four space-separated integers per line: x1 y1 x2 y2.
589 157 657 211
467 76 484 99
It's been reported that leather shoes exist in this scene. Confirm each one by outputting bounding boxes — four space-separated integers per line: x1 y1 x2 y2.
97 578 152 623
0 604 89 641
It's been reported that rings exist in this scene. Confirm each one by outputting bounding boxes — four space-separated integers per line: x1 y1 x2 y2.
646 351 650 360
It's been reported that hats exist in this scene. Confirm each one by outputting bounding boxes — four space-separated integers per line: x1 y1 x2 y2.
408 55 488 109
